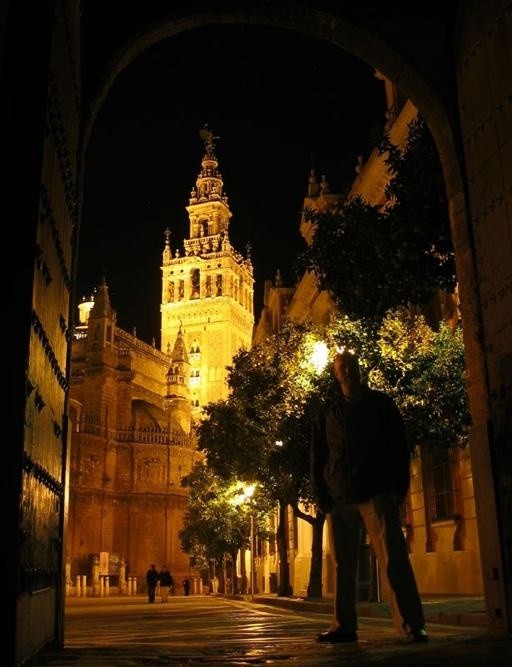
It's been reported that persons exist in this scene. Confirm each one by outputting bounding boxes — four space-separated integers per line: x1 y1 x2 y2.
183 576 190 596
307 354 431 643
158 565 174 602
147 563 160 602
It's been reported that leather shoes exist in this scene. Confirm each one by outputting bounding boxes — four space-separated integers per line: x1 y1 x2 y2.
317 631 358 643
408 628 428 642
